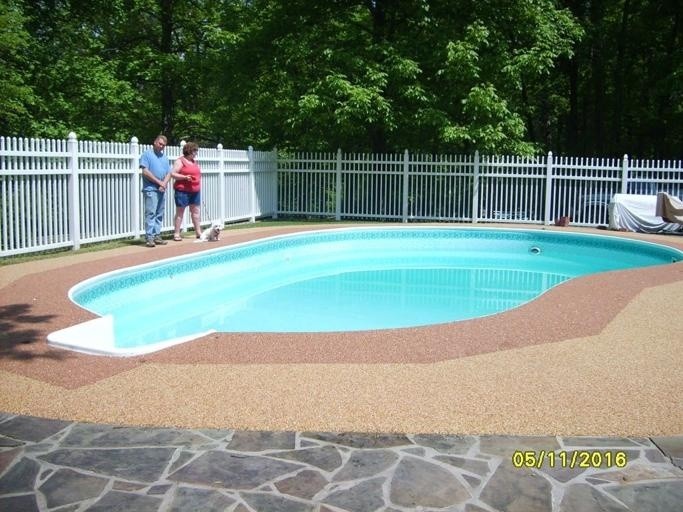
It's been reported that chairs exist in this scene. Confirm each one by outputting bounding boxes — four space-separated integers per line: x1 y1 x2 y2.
654 189 682 228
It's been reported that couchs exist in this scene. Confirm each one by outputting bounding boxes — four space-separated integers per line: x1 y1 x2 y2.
607 192 682 236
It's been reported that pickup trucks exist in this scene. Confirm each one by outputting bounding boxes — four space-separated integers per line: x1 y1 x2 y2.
580 177 683 224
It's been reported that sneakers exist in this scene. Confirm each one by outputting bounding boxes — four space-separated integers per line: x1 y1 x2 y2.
146 236 167 248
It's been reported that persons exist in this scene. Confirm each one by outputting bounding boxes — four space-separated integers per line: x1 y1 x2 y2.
138 134 172 247
169 142 203 242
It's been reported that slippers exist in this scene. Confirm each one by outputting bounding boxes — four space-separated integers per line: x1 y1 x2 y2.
173 234 183 241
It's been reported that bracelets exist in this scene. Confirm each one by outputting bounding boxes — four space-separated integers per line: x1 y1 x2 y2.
183 175 189 180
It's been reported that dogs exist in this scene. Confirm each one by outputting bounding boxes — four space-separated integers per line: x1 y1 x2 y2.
199 219 224 242
